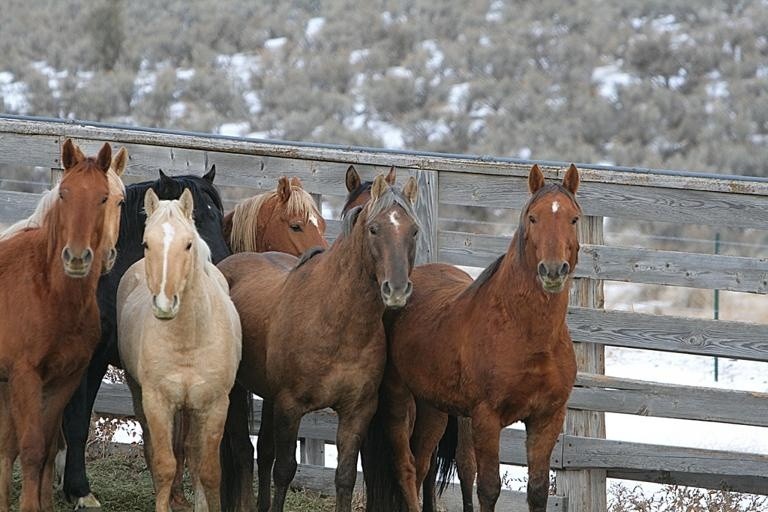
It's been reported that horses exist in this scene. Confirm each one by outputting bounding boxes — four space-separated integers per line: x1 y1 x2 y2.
0 138 583 512
